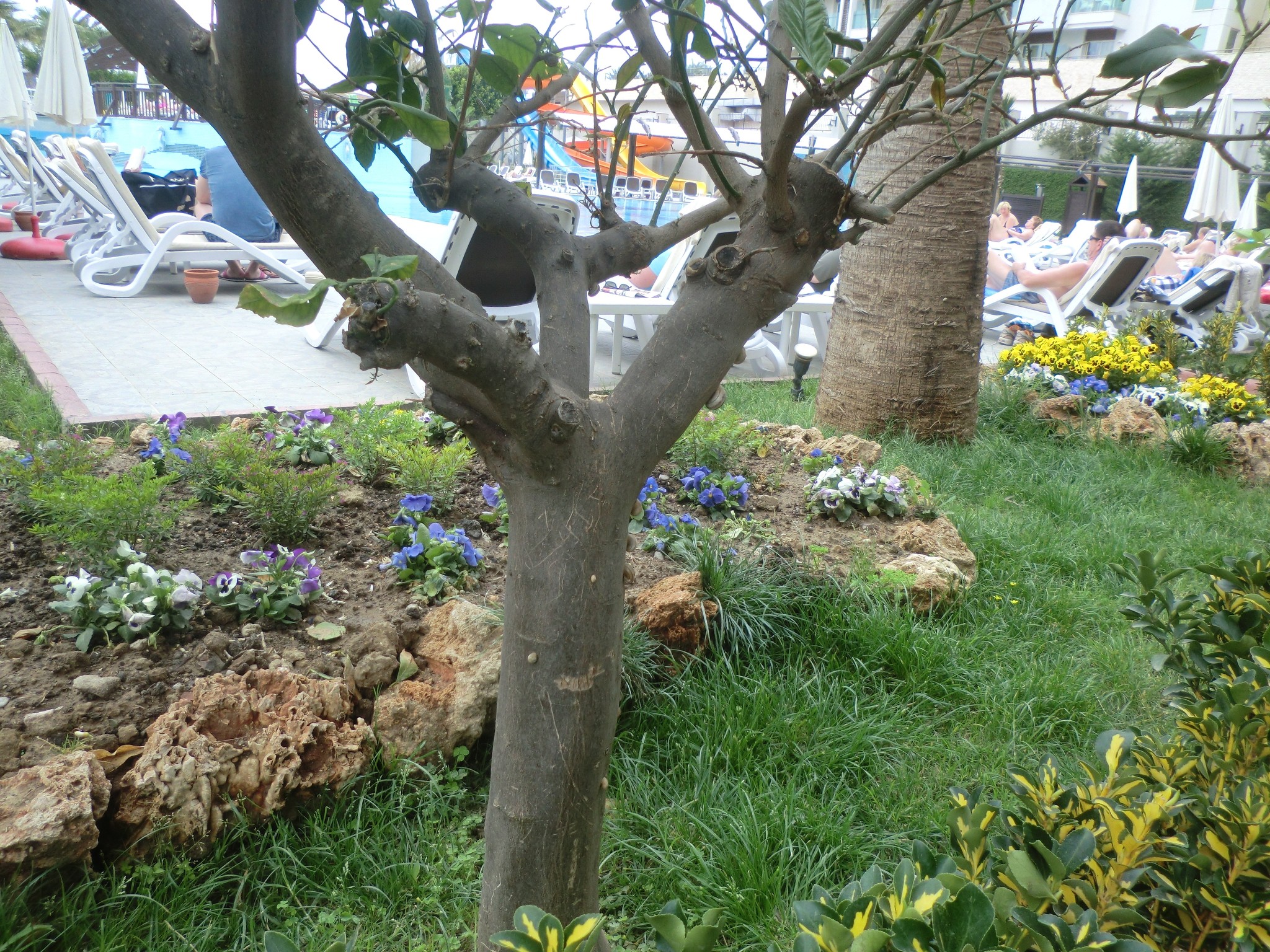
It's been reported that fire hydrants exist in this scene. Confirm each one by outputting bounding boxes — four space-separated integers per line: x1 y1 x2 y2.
788 343 818 402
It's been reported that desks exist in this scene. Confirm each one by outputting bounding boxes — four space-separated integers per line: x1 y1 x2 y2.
585 285 679 388
774 285 846 369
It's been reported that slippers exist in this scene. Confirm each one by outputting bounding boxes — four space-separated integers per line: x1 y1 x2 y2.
245 268 270 282
260 267 279 278
218 269 244 281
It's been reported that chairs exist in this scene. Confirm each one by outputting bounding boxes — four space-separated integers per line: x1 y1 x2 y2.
599 196 785 389
485 165 719 203
982 219 1270 356
302 190 581 400
0 129 316 299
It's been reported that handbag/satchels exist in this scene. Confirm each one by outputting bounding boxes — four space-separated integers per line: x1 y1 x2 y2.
123 167 198 220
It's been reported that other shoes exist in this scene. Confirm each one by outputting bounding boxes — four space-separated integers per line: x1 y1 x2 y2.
1005 320 1033 330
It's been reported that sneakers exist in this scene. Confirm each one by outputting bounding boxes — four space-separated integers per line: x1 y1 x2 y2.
1012 330 1036 345
999 326 1021 345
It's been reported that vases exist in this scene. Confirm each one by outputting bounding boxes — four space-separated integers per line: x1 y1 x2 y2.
182 266 221 305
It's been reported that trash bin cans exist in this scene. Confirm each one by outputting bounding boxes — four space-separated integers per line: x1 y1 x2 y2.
1062 174 1108 234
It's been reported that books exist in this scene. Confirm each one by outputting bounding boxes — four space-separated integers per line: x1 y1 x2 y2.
125 146 146 171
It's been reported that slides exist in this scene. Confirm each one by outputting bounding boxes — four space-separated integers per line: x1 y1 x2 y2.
519 75 674 189
568 74 707 198
514 97 607 188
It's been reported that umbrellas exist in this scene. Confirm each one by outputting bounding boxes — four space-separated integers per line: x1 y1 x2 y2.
0 0 151 216
1116 89 1258 260
522 141 533 168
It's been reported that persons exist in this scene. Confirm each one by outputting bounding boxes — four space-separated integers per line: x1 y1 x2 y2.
987 201 1252 349
627 189 737 289
64 97 284 283
500 163 532 178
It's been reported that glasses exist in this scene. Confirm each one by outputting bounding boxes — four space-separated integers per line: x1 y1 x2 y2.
1090 235 1101 241
603 280 632 291
